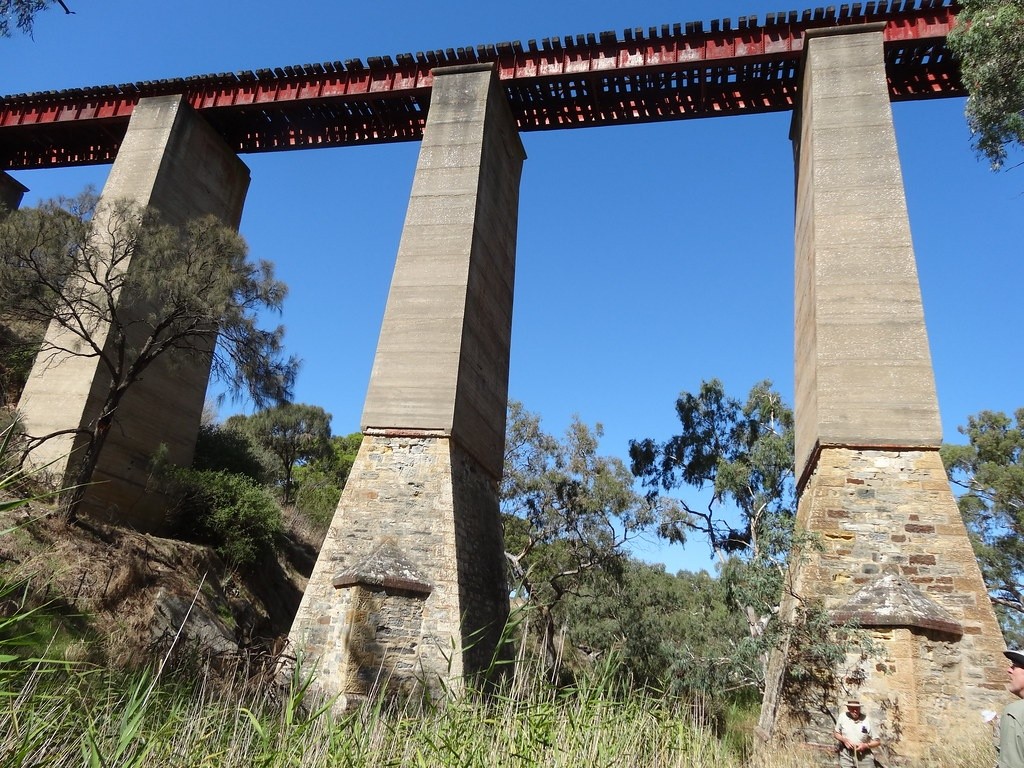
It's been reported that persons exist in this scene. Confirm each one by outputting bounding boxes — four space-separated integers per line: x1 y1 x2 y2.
833 700 881 768
981 709 1001 768
999 647 1024 768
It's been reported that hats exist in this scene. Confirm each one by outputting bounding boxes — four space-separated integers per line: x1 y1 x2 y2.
846 700 864 707
1003 649 1024 665
